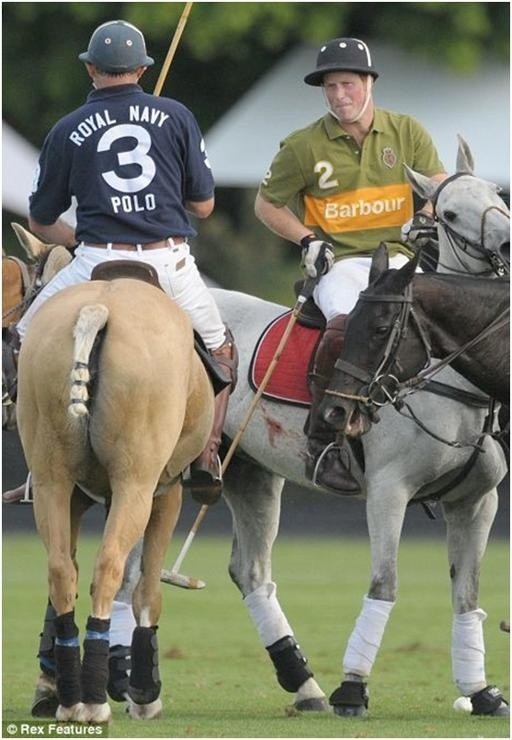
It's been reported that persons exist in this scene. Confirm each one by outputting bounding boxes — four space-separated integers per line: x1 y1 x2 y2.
254 39 451 495
2 18 237 505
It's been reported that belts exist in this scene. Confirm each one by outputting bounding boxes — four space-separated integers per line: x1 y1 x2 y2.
83 234 185 252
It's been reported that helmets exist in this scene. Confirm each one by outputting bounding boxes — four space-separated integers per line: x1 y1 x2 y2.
76 20 157 72
303 36 379 88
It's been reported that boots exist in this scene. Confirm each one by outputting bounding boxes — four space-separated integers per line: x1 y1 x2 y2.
305 312 362 495
189 340 234 505
2 472 32 502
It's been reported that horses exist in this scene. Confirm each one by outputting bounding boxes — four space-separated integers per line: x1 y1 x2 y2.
10 220 216 726
106 131 510 716
316 241 510 440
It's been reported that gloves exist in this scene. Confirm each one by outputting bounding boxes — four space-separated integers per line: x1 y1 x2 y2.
401 210 436 248
301 232 335 278
